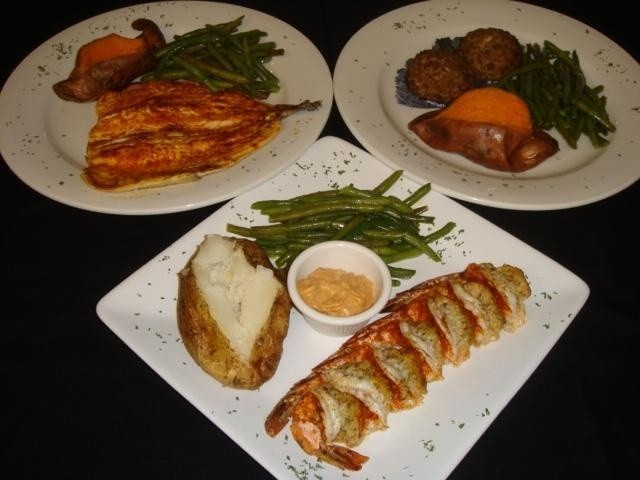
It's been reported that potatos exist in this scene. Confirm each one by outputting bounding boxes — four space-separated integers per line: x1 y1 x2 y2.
177 234 293 390
408 86 560 174
53 18 168 103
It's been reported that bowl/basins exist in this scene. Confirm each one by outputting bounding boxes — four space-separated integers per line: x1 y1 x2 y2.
286 240 393 337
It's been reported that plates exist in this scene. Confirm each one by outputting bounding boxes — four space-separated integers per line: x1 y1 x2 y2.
332 1 640 212
97 134 593 479
2 1 331 216
